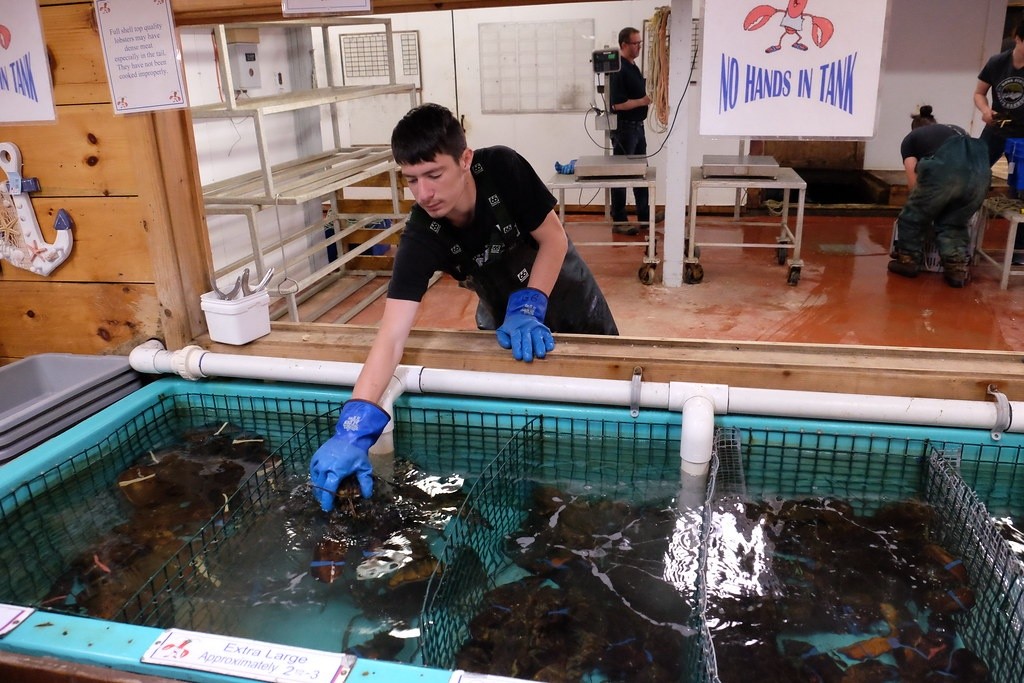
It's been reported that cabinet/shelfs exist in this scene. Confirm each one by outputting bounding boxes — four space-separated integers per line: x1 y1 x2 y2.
176 16 443 325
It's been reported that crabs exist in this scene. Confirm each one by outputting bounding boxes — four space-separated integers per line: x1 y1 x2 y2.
40 421 283 630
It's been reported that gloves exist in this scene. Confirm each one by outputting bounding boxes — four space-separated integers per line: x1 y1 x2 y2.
309 398 391 511
496 287 554 363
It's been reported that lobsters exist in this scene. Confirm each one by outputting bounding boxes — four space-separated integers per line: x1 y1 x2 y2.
291 455 495 660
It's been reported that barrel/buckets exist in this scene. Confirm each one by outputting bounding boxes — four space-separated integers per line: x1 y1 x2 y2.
200 283 270 345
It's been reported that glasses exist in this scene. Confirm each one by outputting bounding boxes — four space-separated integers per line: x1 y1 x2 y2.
624 41 644 46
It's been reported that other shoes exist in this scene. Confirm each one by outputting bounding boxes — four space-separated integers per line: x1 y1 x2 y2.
889 259 918 277
612 225 639 235
944 274 963 288
641 210 665 228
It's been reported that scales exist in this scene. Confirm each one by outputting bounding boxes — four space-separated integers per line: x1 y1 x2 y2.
574 44 648 182
700 136 780 180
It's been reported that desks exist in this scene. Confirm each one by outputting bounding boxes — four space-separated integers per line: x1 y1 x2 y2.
547 167 660 285
683 167 807 286
973 198 1024 291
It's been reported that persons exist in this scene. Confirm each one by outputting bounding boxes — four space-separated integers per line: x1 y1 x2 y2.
609 28 664 238
973 23 1024 268
308 101 620 513
889 105 993 289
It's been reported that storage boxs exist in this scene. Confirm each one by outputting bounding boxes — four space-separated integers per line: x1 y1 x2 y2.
200 284 272 345
0 353 143 462
